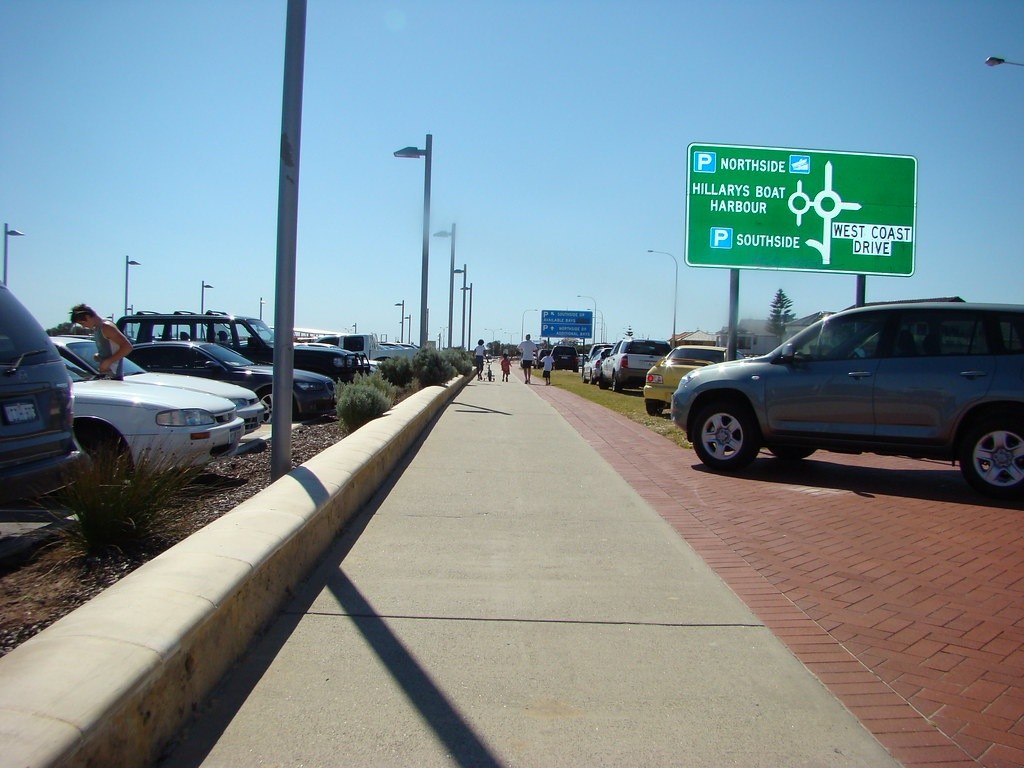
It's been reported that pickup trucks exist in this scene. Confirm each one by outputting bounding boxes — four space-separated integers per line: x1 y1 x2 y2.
315 335 421 362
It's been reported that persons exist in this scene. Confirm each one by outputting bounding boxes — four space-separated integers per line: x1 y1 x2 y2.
517 334 538 384
578 354 588 366
538 350 555 386
472 339 489 380
69 304 133 381
501 353 512 382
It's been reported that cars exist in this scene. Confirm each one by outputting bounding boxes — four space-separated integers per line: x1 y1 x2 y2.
671 301 1024 499
520 340 671 392
0 285 382 505
643 345 746 416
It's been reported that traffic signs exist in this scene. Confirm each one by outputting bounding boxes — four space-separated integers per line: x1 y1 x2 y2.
684 143 918 278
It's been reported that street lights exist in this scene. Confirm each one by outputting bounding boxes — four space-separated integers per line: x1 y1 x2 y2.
648 250 678 348
485 329 518 360
352 303 411 345
394 146 433 385
454 269 466 352
460 287 472 353
125 261 141 335
201 284 267 320
4 230 24 288
433 231 456 348
577 296 607 343
522 310 538 341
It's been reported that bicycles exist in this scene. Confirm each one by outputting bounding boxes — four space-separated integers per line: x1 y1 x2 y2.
483 360 496 381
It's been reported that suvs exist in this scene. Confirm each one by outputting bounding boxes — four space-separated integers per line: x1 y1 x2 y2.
117 311 370 382
